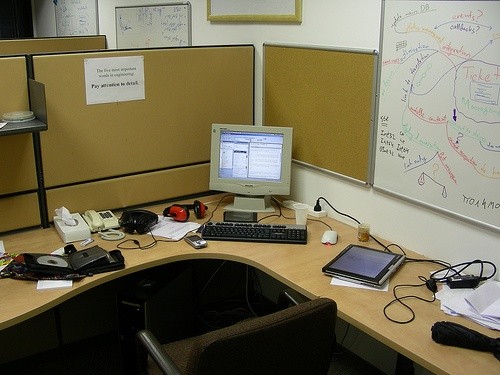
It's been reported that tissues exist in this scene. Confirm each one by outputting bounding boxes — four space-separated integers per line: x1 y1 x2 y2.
54 206 90 243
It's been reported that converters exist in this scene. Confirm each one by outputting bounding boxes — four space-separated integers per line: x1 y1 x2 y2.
446 274 478 288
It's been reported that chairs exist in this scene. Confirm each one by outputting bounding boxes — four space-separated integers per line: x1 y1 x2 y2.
139 288 337 375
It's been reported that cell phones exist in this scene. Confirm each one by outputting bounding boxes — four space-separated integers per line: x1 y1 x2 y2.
184 235 207 248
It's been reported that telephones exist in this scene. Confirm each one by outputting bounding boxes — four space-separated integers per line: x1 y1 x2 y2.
82 210 125 241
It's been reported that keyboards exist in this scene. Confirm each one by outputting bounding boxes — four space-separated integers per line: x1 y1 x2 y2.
201 222 307 244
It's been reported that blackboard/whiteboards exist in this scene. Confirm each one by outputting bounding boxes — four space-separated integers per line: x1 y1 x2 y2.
54 0 99 37
115 1 192 49
373 0 500 231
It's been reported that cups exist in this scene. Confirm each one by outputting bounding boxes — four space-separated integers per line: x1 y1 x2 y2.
358 223 370 242
294 203 309 224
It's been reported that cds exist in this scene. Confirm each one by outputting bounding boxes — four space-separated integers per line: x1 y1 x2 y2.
36 255 68 267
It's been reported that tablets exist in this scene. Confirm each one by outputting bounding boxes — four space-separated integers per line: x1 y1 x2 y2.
322 244 406 289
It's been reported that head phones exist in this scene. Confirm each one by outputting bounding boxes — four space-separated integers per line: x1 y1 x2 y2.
163 200 208 222
118 210 158 234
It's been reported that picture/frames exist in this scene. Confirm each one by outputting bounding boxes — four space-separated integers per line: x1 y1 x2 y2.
207 0 302 23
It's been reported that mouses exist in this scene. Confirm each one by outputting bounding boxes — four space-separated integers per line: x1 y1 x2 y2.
321 230 338 244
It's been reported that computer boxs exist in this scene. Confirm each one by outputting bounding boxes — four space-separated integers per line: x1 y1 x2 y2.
113 277 177 375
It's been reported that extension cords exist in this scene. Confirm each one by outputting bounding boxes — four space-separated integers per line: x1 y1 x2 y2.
283 199 326 218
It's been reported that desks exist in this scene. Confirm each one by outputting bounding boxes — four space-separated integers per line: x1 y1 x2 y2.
0 191 499 375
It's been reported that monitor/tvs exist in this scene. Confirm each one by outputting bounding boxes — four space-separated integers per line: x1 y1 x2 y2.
208 123 294 195
